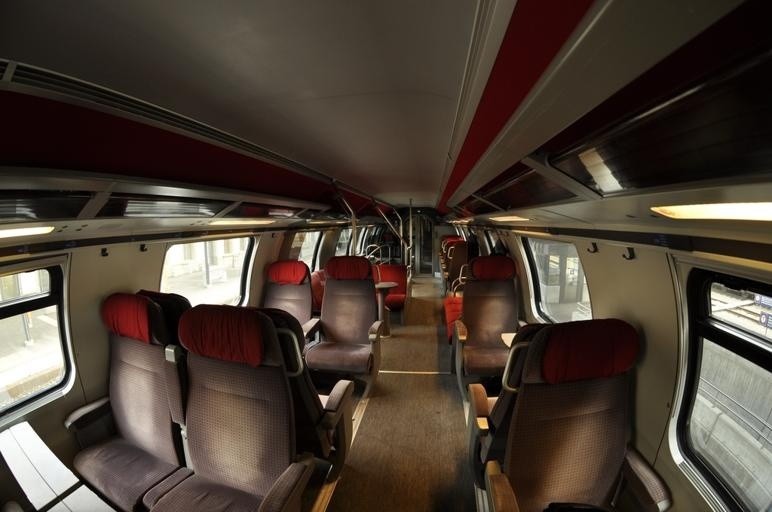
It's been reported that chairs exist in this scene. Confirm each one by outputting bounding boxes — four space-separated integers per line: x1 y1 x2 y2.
438 235 675 511
63 253 409 511
